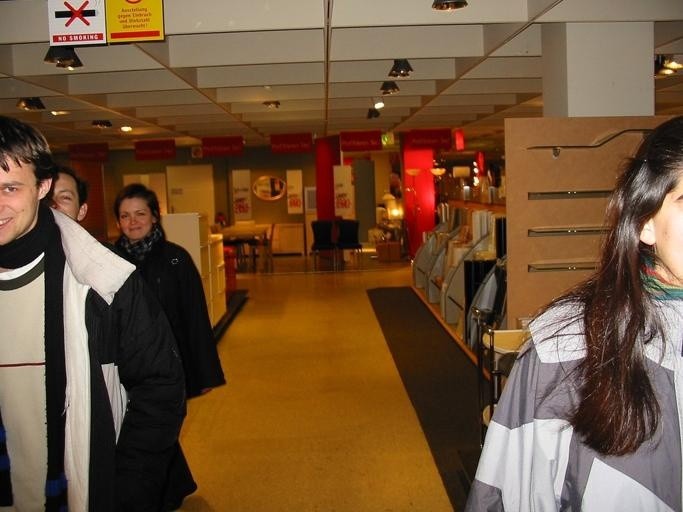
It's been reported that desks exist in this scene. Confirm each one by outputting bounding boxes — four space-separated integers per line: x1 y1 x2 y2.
218 225 267 273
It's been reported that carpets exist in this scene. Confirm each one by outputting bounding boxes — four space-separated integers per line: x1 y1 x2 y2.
366 285 489 512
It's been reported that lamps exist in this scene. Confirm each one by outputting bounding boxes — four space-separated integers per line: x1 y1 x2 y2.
43 46 83 67
17 97 46 112
379 60 414 96
432 0 468 9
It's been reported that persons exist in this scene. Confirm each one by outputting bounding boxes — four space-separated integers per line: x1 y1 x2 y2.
370 226 395 258
113 184 226 512
0 116 188 512
51 165 88 223
465 117 683 512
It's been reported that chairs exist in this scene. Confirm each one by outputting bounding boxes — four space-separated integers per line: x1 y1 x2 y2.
309 219 363 272
249 223 274 266
210 222 246 267
